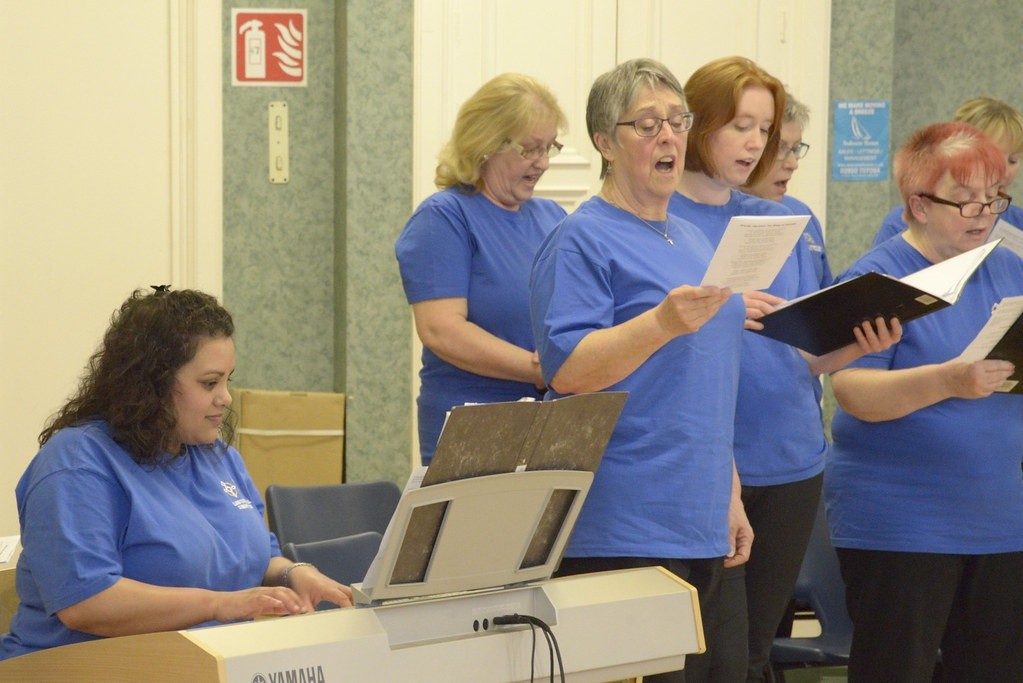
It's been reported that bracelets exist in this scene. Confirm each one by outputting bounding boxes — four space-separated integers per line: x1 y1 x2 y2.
281 563 311 587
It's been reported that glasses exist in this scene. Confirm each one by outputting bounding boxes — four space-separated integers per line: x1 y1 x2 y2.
776 142 809 161
503 137 564 161
616 112 694 137
918 191 1012 218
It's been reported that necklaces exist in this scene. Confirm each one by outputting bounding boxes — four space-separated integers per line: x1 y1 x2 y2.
629 210 673 245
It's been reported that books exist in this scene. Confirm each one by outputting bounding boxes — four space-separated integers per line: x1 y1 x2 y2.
744 239 1004 357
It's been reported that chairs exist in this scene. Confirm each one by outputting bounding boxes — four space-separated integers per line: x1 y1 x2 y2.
265 480 402 611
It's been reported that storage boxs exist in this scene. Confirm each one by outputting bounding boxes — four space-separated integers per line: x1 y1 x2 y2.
232 387 345 526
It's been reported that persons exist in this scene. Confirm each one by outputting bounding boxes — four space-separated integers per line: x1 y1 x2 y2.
821 96 1023 683
0 287 354 662
668 56 904 683
745 92 832 292
394 73 570 465
529 58 754 683
